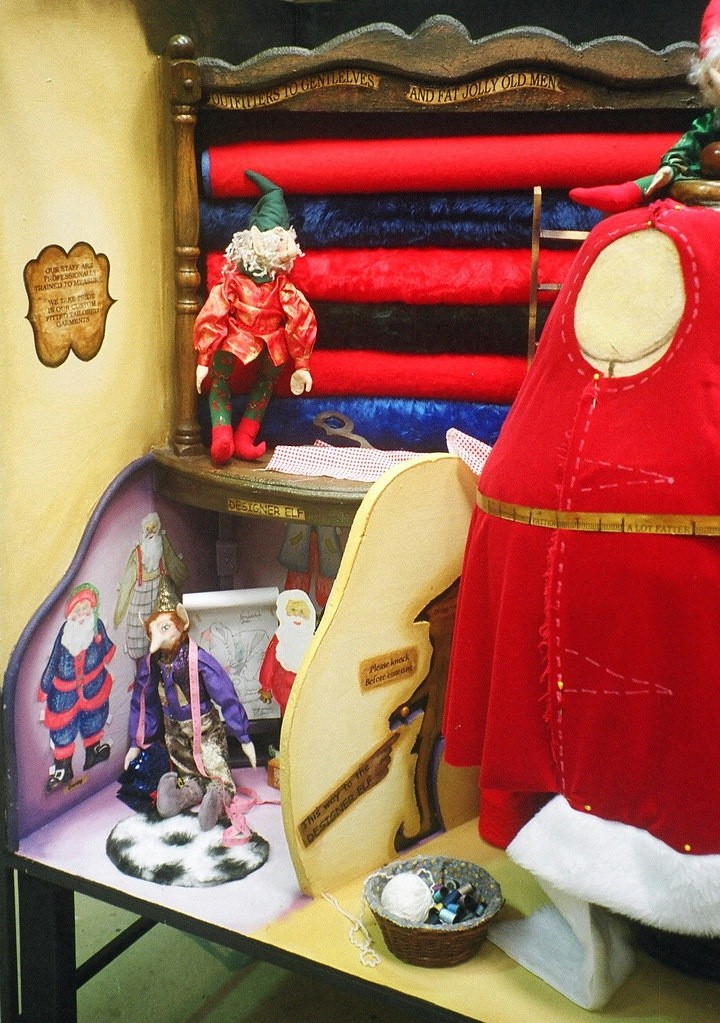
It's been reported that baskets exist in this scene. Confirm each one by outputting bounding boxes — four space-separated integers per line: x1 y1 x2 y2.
364 855 505 968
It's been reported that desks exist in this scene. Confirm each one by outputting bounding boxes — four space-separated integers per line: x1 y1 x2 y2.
16 765 720 1023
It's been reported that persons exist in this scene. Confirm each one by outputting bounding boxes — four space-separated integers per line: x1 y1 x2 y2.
116 601 255 831
190 226 316 469
